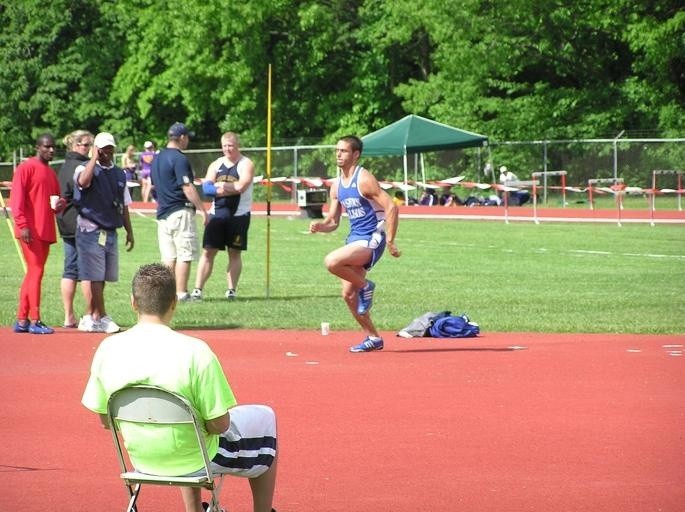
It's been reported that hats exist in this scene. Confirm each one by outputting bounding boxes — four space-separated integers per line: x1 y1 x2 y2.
94 132 117 149
500 166 508 173
169 122 193 136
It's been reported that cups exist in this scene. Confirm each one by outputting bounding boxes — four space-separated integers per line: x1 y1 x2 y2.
50 196 59 210
321 322 330 336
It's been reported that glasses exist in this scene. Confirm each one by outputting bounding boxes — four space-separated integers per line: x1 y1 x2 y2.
77 142 93 147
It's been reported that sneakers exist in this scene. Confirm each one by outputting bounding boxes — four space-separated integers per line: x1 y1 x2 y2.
15 320 30 332
350 334 384 353
78 317 99 332
176 292 190 301
29 321 55 334
357 280 376 315
226 289 235 298
191 288 202 299
98 316 120 333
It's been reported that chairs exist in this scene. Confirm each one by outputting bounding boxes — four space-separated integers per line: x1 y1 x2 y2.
107 384 224 512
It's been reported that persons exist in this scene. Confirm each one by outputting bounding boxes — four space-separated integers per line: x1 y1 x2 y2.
9 132 61 336
499 166 521 183
308 135 403 353
120 144 135 194
136 141 155 205
73 131 136 335
51 129 91 329
150 123 209 300
81 264 279 511
190 132 254 299
390 186 500 210
